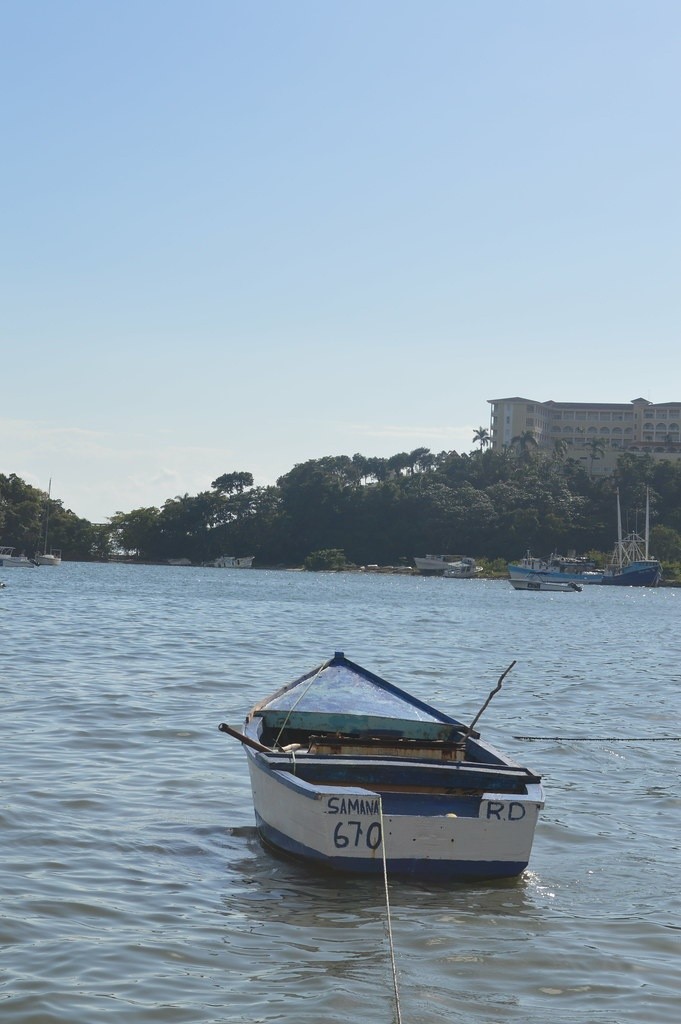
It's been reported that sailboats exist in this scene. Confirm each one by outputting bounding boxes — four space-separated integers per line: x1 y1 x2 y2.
602 483 664 587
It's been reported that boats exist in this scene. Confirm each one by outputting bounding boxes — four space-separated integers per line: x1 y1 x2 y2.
507 547 605 585
0 478 62 568
414 554 484 578
201 554 255 569
242 652 545 881
508 572 583 592
168 558 195 566
